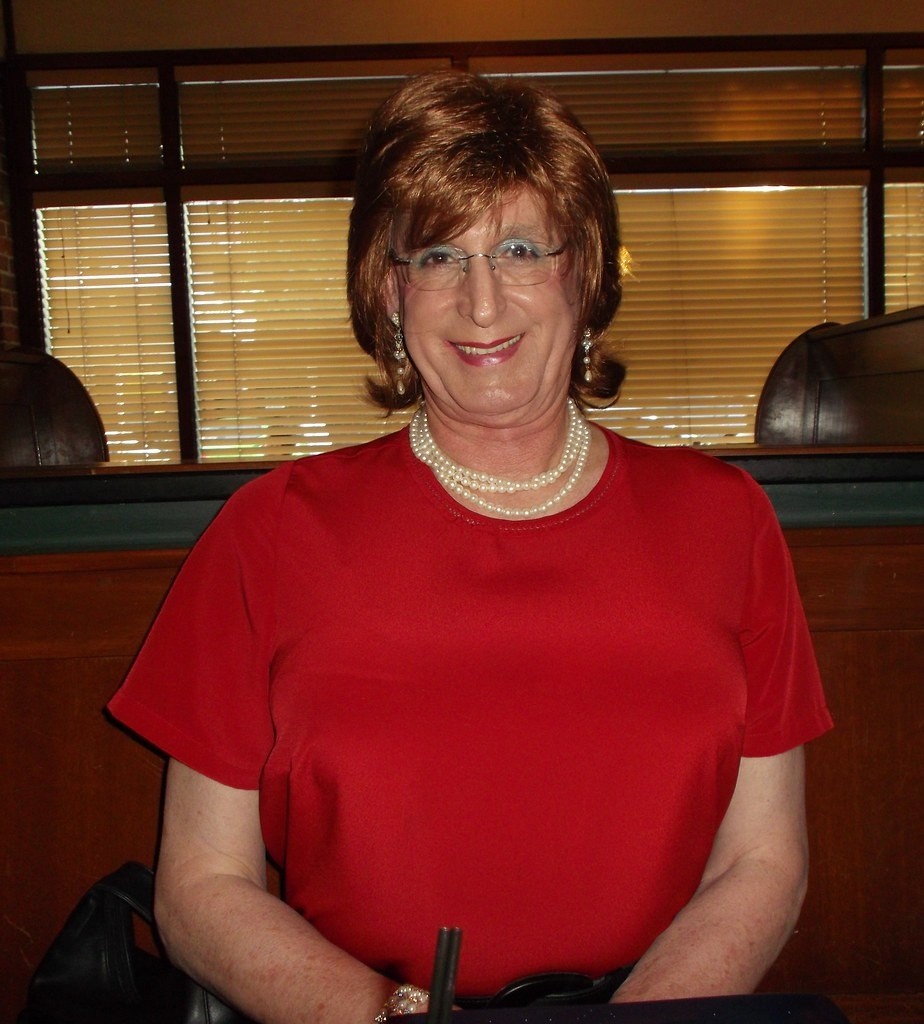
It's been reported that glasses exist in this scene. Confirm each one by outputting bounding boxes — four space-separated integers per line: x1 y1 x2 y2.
389 237 570 292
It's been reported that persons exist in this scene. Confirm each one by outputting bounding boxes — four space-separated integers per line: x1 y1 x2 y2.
103 66 837 1024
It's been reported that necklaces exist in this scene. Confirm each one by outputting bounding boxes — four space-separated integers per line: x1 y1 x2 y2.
409 395 593 517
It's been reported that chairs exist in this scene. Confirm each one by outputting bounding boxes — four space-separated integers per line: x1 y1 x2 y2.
743 301 924 455
0 344 116 470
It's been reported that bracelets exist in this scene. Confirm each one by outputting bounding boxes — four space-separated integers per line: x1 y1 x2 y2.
375 983 430 1022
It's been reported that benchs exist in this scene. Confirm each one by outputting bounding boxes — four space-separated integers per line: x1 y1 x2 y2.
0 477 921 1016
